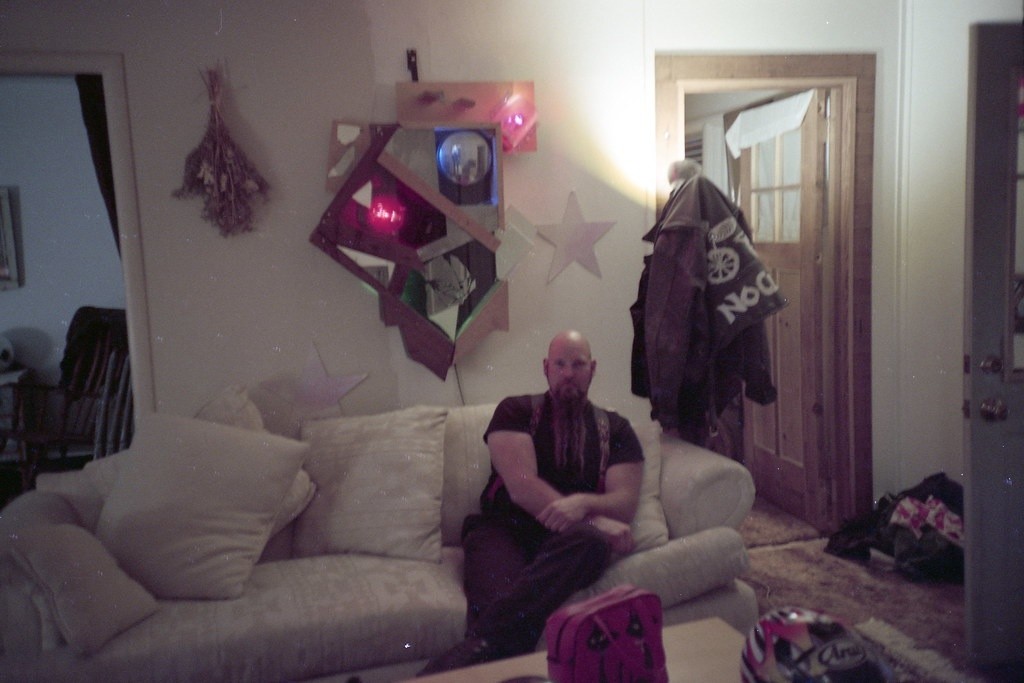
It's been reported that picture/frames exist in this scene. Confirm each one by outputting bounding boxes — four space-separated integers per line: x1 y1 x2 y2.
0 185 20 288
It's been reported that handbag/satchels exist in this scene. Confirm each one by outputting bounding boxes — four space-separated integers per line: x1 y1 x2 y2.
544 583 670 683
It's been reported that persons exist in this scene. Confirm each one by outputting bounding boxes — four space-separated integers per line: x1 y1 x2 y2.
415 331 646 679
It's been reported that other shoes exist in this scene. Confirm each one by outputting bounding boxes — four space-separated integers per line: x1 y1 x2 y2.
416 635 489 676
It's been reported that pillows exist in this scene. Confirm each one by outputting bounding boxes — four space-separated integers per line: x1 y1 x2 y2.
293 405 450 564
1 524 157 658
97 413 312 598
85 387 317 542
621 420 671 556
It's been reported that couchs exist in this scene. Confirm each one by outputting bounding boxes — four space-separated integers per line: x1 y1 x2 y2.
0 405 753 683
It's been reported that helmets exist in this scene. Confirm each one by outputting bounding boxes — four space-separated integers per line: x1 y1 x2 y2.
740 605 892 683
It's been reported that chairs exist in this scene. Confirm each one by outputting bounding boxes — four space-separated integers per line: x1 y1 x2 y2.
0 307 131 484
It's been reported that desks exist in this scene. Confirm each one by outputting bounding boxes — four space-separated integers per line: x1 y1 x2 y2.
0 367 32 463
404 617 745 683
